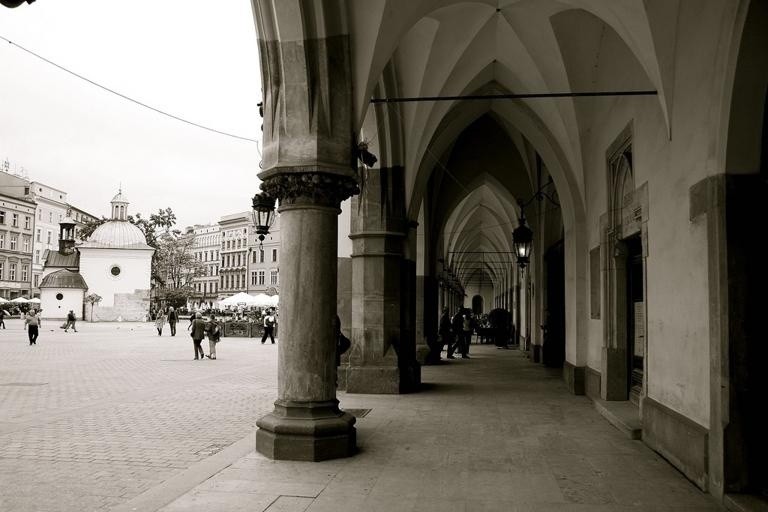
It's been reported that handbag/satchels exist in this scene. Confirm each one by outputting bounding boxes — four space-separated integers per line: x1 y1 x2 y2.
265 319 274 326
338 333 350 354
210 330 221 340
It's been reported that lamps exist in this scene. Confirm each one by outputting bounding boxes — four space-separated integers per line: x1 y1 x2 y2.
251 191 276 252
511 191 560 278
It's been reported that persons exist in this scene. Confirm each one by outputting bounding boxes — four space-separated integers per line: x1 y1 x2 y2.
439 306 475 359
261 311 275 344
335 315 342 388
167 306 179 336
64 310 78 333
154 309 166 336
0 305 41 346
187 309 220 360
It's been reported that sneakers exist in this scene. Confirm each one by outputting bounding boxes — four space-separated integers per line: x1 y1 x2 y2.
194 353 217 359
446 353 470 359
